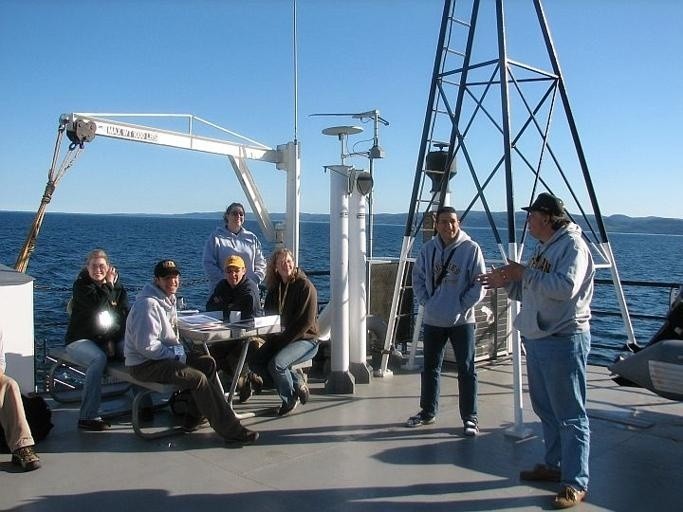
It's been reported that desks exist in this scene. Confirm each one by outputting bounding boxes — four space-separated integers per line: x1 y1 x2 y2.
172 328 280 433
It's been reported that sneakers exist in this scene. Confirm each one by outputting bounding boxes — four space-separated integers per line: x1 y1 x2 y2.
518 463 562 484
403 411 437 427
78 417 111 433
182 414 210 432
223 424 259 450
550 484 590 510
238 372 264 404
462 418 479 437
10 445 42 473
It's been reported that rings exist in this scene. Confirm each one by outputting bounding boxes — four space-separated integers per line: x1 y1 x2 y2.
503 275 506 280
501 269 505 273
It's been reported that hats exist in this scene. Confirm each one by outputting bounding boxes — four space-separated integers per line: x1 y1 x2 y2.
520 193 564 214
153 259 181 278
223 254 245 269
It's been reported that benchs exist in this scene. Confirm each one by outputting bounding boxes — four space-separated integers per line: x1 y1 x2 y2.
43 347 188 442
287 357 313 391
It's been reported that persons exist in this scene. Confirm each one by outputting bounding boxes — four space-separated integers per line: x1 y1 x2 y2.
64 248 153 429
123 260 260 443
264 247 320 417
206 256 261 403
405 207 488 436
204 202 267 298
0 325 41 471
476 193 596 509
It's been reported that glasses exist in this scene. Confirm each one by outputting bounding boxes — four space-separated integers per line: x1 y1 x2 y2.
230 210 243 217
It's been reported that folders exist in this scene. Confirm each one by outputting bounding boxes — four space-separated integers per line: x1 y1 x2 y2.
229 314 281 329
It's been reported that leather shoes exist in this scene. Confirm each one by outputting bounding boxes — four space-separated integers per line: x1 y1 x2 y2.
277 368 308 417
